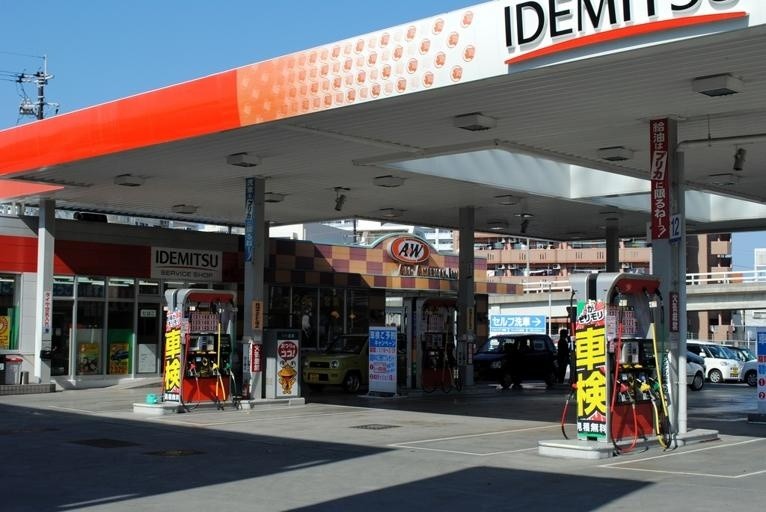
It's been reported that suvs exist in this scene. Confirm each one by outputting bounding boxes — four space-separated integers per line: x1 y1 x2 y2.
299 333 370 393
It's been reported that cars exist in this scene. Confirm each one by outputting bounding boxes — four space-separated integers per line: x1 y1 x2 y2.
111 349 129 361
82 351 98 364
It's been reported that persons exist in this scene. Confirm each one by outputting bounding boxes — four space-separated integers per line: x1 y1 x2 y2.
556 329 570 383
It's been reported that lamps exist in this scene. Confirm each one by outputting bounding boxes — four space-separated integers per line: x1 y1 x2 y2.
334 188 349 211
691 77 745 98
598 147 635 162
171 205 199 214
485 220 508 233
380 209 404 219
263 191 286 203
707 174 738 186
225 153 261 167
513 213 534 233
732 144 746 170
373 177 404 187
495 195 522 208
113 176 145 186
452 116 498 131
600 212 621 222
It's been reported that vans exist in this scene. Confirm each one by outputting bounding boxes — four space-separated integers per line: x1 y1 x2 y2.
471 331 559 390
684 337 758 392
